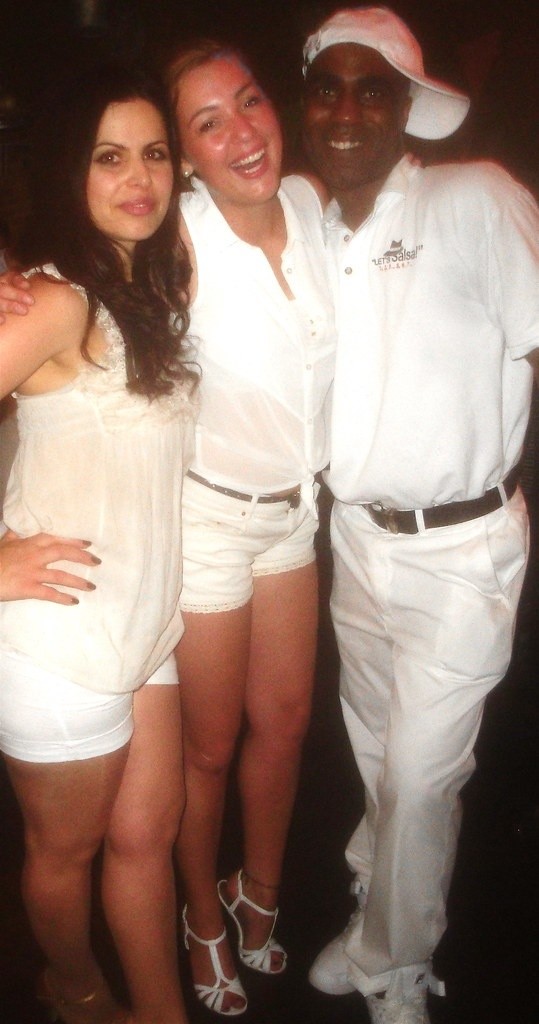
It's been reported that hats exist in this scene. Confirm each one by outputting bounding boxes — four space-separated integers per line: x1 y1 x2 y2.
301 7 470 140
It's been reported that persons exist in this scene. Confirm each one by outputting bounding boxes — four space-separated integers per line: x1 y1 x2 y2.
295 6 539 1024
0 8 539 1024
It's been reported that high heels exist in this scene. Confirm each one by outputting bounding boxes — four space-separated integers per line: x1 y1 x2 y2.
181 902 249 1019
217 868 288 975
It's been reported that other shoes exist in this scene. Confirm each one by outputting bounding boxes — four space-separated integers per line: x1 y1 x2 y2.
367 964 432 1023
308 892 370 995
36 965 128 1024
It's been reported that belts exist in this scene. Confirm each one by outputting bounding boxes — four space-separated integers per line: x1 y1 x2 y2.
361 467 519 535
186 469 302 510
